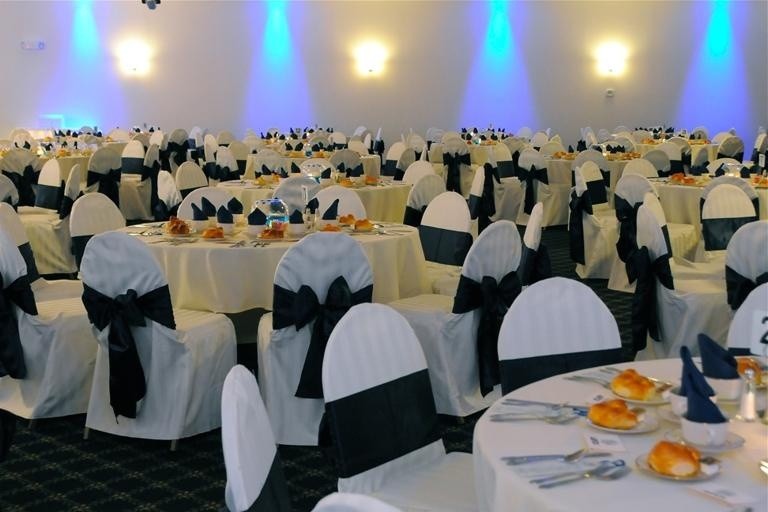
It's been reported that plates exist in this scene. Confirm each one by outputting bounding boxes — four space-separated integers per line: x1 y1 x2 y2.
701 355 767 405
635 452 720 483
663 430 746 451
658 403 687 424
585 413 659 435
610 378 671 405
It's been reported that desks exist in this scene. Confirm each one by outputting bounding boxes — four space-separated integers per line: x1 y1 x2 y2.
470 356 768 512
114 219 433 369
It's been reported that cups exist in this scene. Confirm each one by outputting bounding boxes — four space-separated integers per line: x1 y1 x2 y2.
670 386 720 417
677 417 732 447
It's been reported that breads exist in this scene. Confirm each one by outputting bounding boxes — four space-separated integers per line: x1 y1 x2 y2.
736 358 762 384
353 218 373 231
587 399 639 430
59 149 66 156
319 223 341 232
290 151 305 158
0 150 8 157
164 215 189 234
270 173 280 182
201 224 224 239
270 221 287 232
366 175 379 186
340 214 356 224
256 229 285 240
646 441 703 478
254 177 267 185
340 178 354 186
610 368 657 403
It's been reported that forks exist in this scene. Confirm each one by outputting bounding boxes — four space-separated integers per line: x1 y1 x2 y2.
127 208 412 248
489 363 661 426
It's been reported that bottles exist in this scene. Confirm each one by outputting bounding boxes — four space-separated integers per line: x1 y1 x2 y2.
742 369 756 423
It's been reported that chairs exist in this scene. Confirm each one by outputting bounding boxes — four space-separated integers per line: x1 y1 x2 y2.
415 190 473 294
1 204 85 300
428 201 545 295
631 208 729 358
319 302 473 512
609 174 719 361
725 282 768 356
568 166 619 280
220 365 396 512
496 276 625 398
77 229 237 453
723 220 768 316
2 232 98 430
256 231 374 447
387 218 524 418
1 114 765 254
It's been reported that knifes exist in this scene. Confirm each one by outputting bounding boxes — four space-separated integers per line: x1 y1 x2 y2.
500 448 632 489
